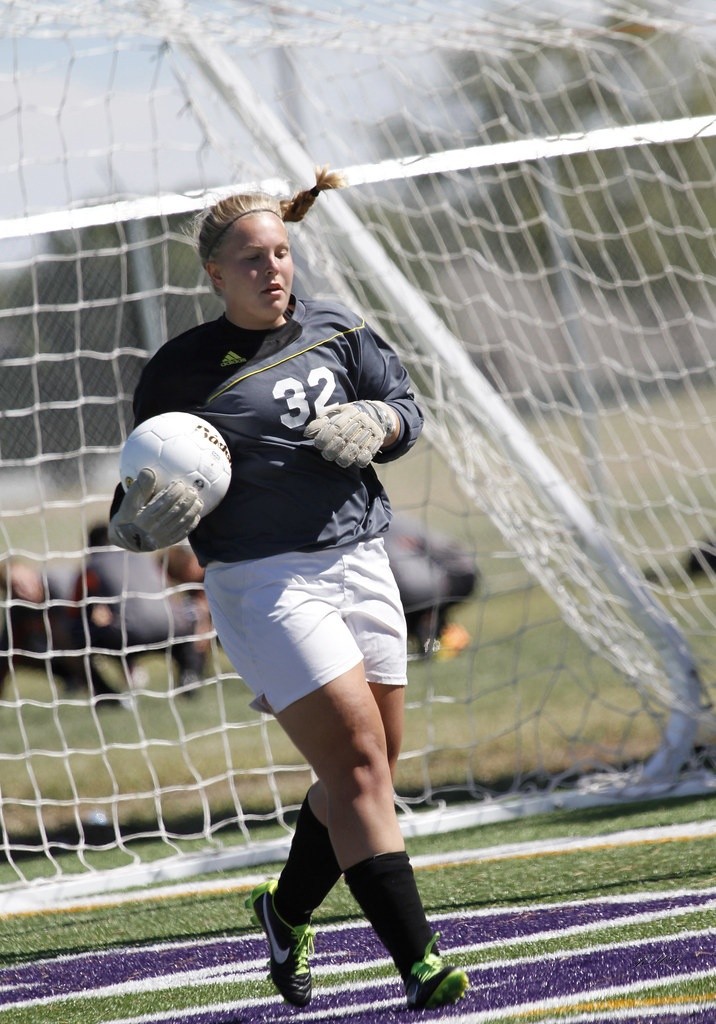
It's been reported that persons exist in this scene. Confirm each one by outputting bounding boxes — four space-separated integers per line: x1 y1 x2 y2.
0 517 482 712
108 169 469 1009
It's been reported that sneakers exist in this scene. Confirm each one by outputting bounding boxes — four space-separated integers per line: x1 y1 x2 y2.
244 877 318 1007
406 931 468 1008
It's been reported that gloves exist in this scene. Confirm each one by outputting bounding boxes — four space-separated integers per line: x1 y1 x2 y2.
304 401 397 467
108 467 206 552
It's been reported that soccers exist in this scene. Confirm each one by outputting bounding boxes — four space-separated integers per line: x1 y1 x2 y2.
117 409 234 518
